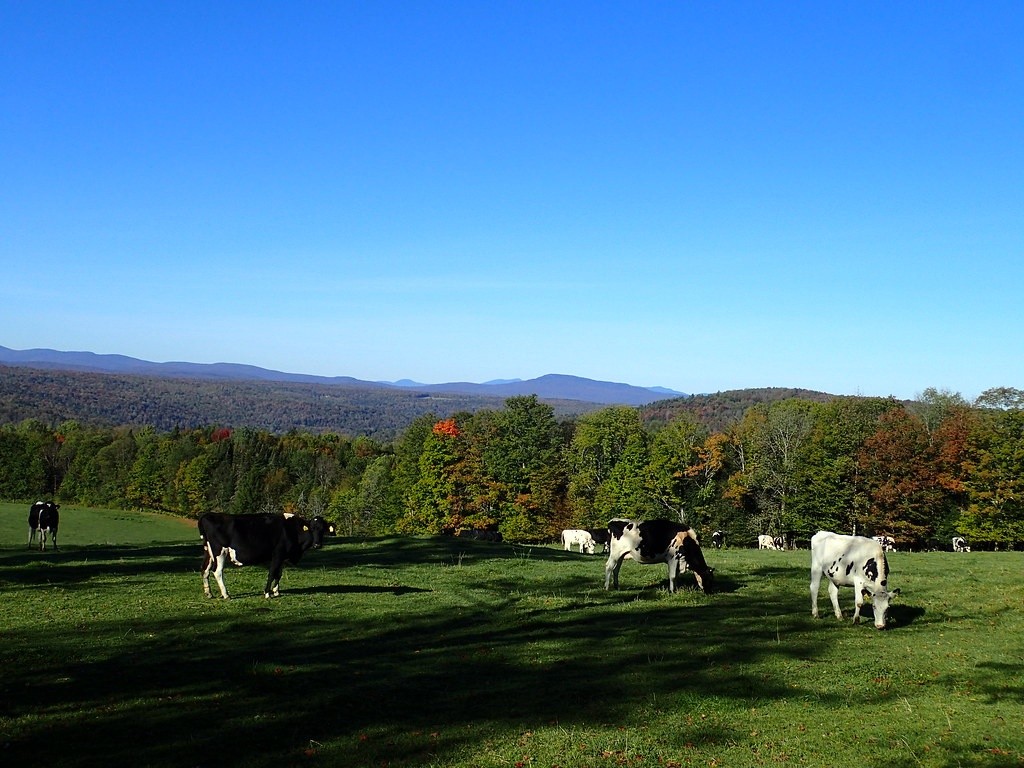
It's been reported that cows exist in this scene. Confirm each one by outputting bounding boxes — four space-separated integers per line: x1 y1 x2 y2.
952 536 971 552
810 530 901 629
758 534 784 551
560 517 728 595
27 500 60 552
197 510 337 598
